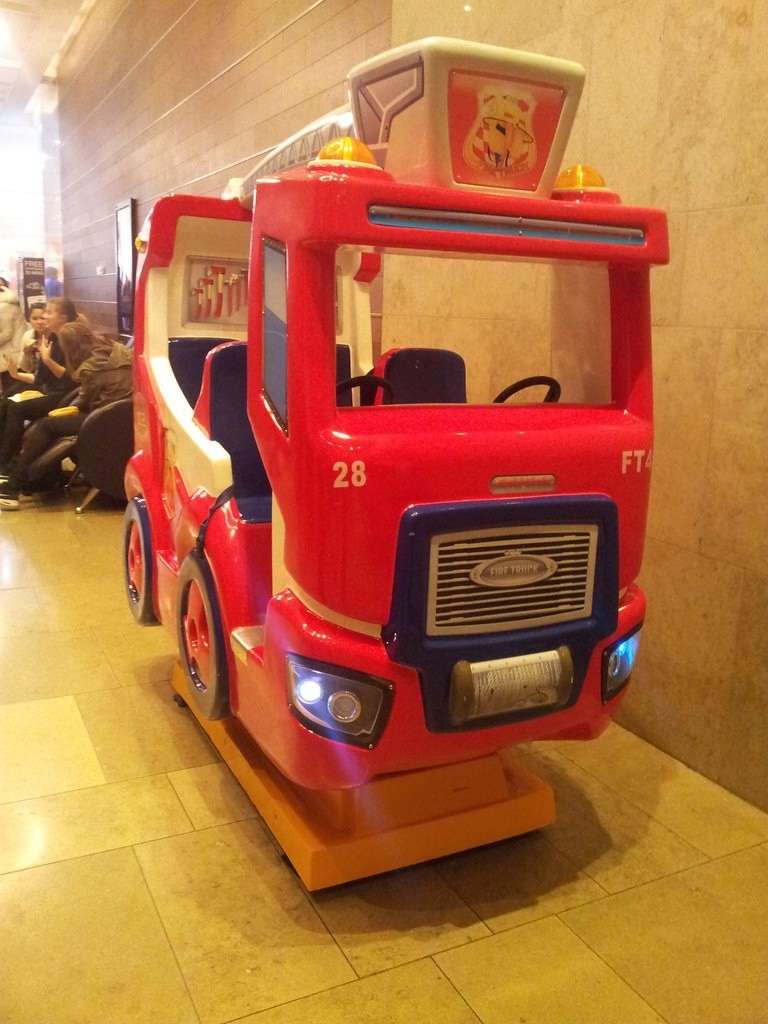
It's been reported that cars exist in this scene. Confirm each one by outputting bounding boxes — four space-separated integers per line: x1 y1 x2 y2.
122 36 672 789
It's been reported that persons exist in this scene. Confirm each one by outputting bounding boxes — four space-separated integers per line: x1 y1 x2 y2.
0 277 133 509
45 267 62 297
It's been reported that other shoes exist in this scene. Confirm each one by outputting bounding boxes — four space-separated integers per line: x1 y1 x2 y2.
0 488 18 508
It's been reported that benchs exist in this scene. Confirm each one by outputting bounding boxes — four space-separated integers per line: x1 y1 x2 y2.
65 395 135 512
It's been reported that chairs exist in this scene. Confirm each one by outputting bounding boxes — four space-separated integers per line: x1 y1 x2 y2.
373 346 468 405
196 340 352 523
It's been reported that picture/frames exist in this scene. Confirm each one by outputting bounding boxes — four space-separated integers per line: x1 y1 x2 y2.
115 199 135 337
